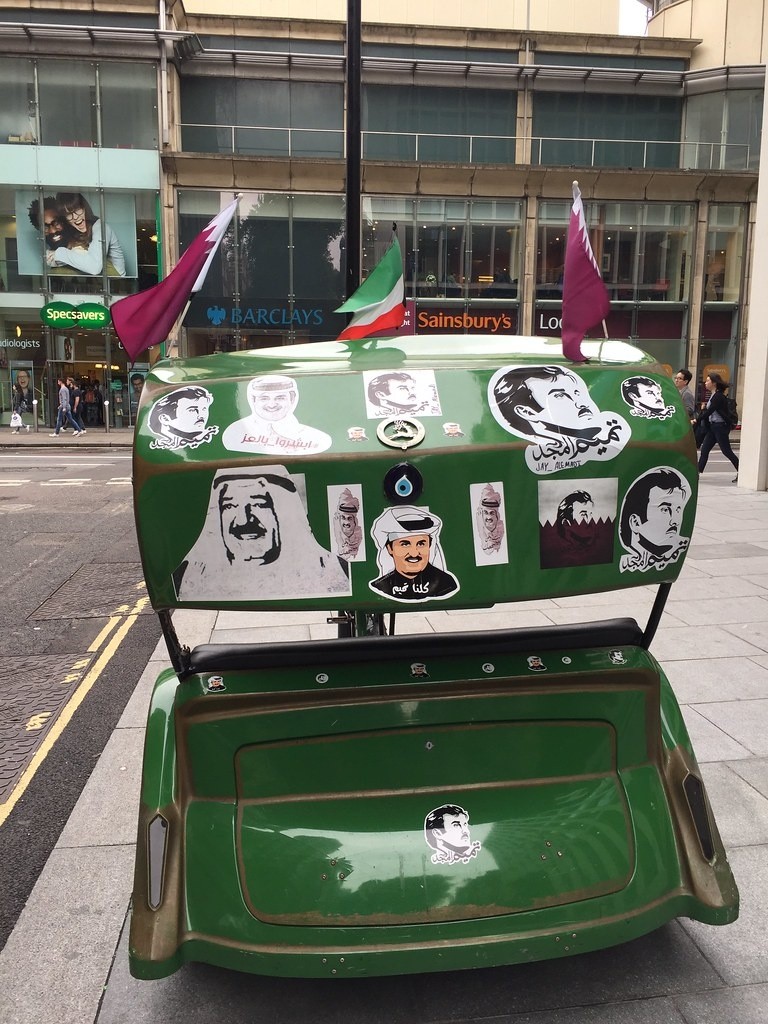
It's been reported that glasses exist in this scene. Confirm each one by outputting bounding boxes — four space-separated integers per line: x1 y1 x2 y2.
44 219 61 232
674 377 685 381
65 207 85 221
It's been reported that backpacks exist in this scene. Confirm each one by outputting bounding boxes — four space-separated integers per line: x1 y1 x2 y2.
727 397 738 429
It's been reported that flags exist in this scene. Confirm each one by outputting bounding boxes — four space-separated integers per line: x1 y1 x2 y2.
333 231 406 340
560 181 610 362
110 193 243 372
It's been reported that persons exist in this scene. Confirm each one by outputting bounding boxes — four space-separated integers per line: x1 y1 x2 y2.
48 377 102 437
10 384 30 434
131 374 144 425
674 369 739 483
28 192 127 276
447 272 457 288
13 370 33 412
63 337 72 361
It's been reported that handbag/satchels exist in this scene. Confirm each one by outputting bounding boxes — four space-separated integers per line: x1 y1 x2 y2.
10 413 23 427
86 391 94 403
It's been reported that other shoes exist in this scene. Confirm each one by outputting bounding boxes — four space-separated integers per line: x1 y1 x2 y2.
84 430 86 434
72 430 78 435
49 433 59 436
12 430 19 434
76 430 83 436
731 473 738 483
25 425 29 432
60 427 66 432
